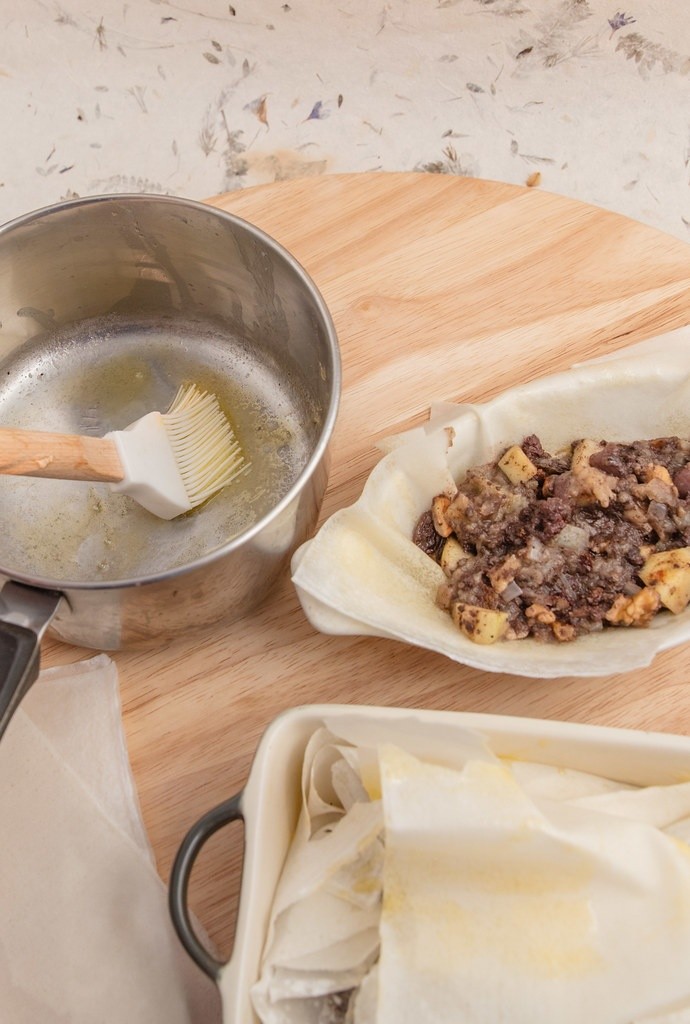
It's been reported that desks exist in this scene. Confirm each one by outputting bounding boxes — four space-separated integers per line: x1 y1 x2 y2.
0 172 690 1023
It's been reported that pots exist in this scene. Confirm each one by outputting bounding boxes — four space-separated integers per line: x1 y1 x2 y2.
0 193 344 742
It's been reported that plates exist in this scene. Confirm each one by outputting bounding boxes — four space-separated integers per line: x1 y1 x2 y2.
169 704 689 1024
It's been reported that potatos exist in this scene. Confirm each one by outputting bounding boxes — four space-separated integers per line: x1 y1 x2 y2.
430 438 690 644
